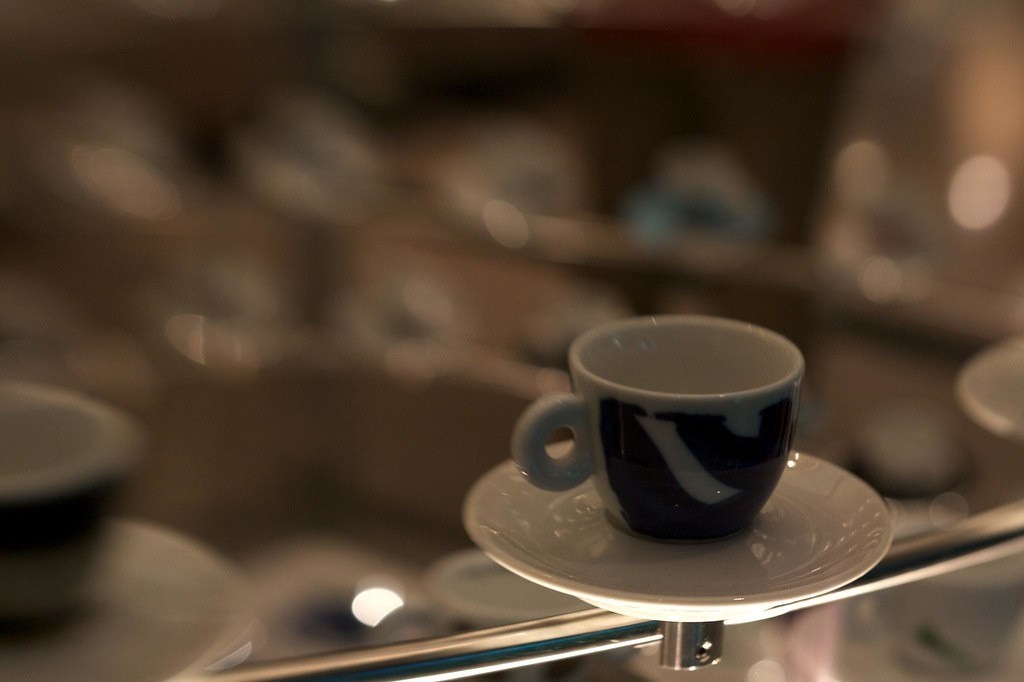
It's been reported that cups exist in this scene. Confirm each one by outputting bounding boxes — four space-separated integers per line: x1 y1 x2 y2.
0 381 151 625
511 314 807 545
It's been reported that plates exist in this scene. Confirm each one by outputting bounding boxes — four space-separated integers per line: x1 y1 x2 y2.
0 519 252 682
952 332 1024 443
462 438 895 622
